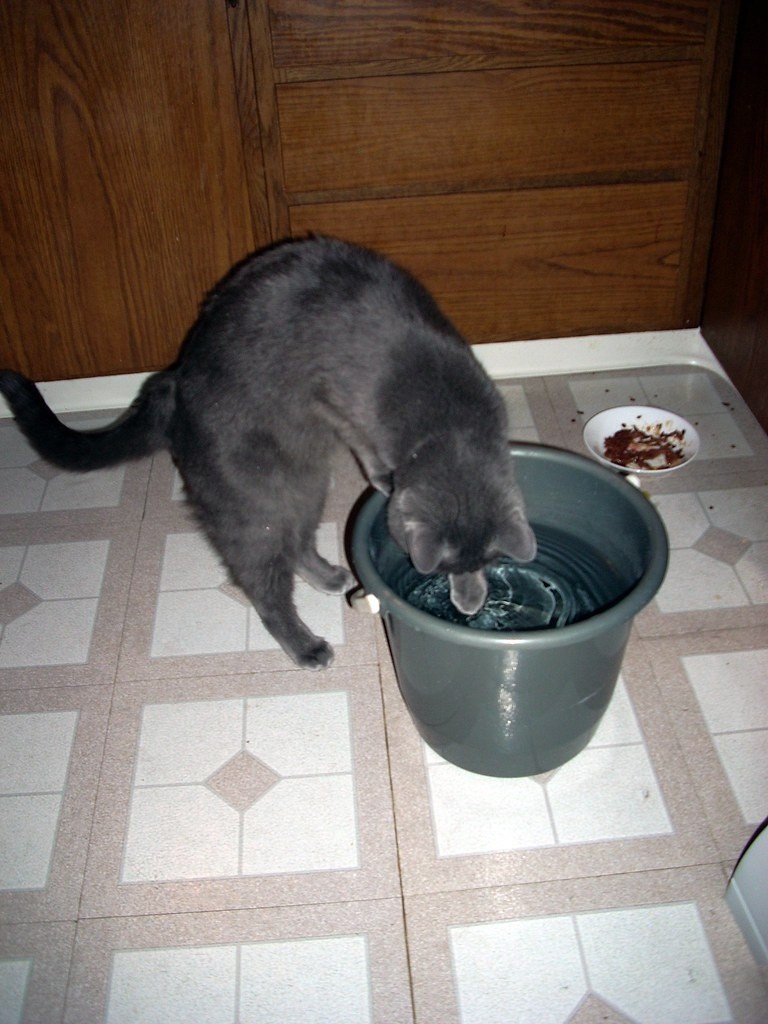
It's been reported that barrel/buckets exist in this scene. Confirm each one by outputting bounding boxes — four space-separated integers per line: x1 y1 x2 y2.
352 440 670 777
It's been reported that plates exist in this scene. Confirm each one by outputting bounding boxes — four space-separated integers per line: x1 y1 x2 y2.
583 406 700 475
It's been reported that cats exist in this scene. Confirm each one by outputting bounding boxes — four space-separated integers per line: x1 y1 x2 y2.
0 232 537 673
